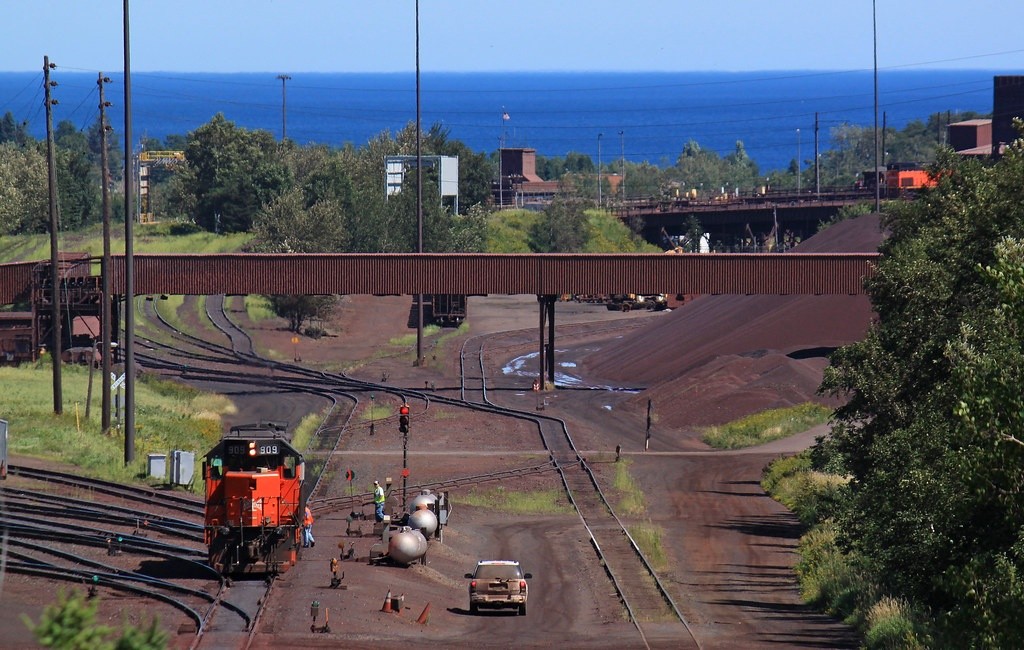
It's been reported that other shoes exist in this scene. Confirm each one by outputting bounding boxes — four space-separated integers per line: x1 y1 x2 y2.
374 522 380 525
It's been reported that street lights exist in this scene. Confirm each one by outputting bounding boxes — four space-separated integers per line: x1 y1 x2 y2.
82 339 119 419
369 393 375 436
617 130 626 200
794 128 802 202
497 112 512 209
275 73 293 138
597 132 605 208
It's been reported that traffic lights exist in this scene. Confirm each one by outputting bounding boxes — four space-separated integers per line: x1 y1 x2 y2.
399 405 410 433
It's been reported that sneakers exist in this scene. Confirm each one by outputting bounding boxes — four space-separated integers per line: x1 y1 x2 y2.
302 544 309 548
311 542 315 547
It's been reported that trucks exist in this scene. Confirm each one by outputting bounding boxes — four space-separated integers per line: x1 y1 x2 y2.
628 294 668 304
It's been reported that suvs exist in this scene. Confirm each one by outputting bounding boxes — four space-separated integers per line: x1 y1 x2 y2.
462 558 533 617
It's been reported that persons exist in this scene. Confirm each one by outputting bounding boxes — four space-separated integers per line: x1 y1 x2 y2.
373 480 385 525
302 505 316 548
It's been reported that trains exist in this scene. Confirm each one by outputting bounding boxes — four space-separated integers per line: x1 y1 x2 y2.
199 422 304 577
860 162 954 197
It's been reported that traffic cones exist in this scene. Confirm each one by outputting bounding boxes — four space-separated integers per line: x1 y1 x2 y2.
380 588 395 613
416 601 432 624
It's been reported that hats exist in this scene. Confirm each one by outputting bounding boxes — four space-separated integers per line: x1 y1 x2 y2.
374 481 379 484
